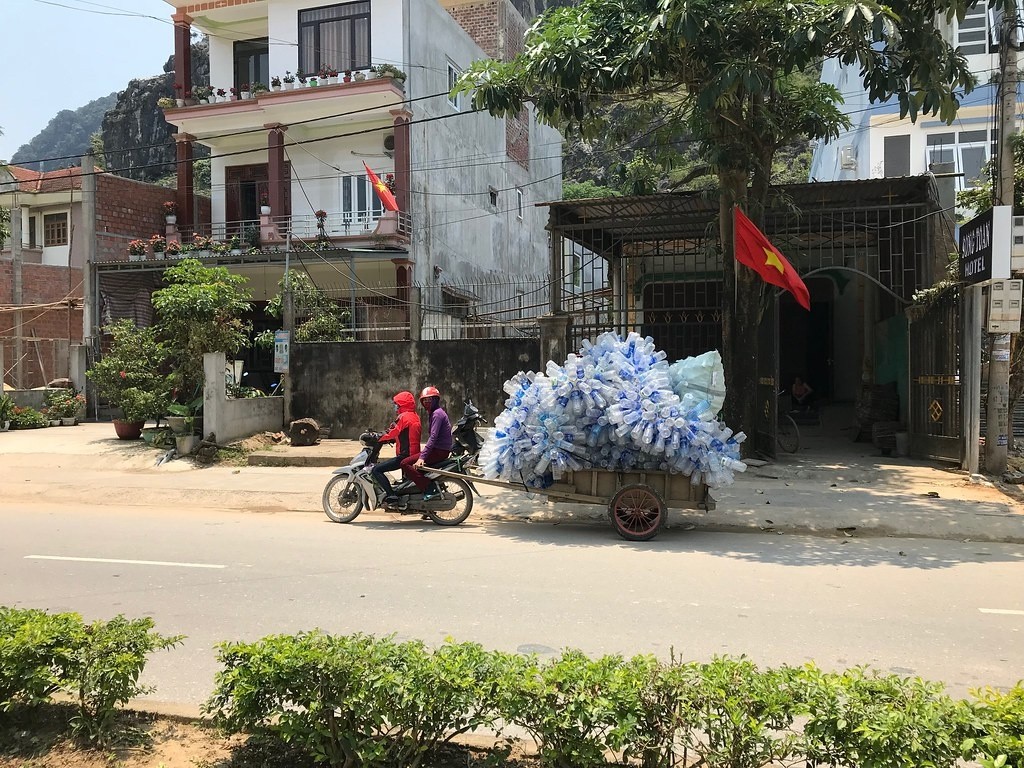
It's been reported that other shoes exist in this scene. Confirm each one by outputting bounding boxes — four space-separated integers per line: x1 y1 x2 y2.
381 497 398 507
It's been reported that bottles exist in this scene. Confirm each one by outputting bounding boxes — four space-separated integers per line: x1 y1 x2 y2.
477 331 747 490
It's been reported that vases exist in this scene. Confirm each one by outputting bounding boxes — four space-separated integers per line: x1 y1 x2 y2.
344 77 350 82
241 91 249 100
218 96 225 102
231 96 237 101
50 420 61 426
129 249 211 260
176 99 186 107
208 96 216 104
262 205 271 214
62 417 76 426
0 420 11 431
329 77 338 84
185 99 196 106
310 81 317 87
167 216 177 225
318 79 329 85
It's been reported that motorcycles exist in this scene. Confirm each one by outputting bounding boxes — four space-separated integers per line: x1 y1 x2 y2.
321 427 478 526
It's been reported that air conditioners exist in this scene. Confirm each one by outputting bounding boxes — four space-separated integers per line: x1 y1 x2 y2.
383 132 395 154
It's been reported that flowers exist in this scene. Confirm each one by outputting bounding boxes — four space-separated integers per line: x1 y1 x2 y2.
344 70 351 76
164 200 177 215
185 89 197 100
205 86 215 97
327 65 339 77
172 83 190 99
127 232 214 255
317 69 329 79
259 190 270 206
217 88 227 96
230 87 239 96
240 83 251 92
0 385 89 430
385 173 396 195
310 78 316 81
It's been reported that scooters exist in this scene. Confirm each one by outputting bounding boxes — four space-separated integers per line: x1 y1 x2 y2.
446 388 488 457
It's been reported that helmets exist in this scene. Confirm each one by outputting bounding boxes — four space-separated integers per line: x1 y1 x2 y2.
419 387 440 406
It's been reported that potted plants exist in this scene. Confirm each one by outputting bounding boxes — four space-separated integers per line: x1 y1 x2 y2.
157 97 177 109
192 85 207 104
212 234 244 257
271 76 282 92
83 317 203 456
354 64 406 84
295 67 306 88
283 71 296 90
250 82 268 97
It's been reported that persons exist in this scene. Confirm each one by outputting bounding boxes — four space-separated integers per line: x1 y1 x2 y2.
363 391 421 506
400 387 452 501
788 375 814 414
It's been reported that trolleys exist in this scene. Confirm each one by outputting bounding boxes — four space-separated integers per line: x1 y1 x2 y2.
415 456 722 541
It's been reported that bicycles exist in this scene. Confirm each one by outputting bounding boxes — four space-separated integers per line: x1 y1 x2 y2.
778 389 802 454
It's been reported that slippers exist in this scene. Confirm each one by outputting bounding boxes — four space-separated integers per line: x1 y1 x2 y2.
423 493 440 501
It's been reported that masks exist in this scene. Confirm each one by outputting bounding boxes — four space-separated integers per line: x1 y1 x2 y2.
394 405 398 412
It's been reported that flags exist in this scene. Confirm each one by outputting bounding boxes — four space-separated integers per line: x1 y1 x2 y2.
734 207 811 310
366 166 399 212
